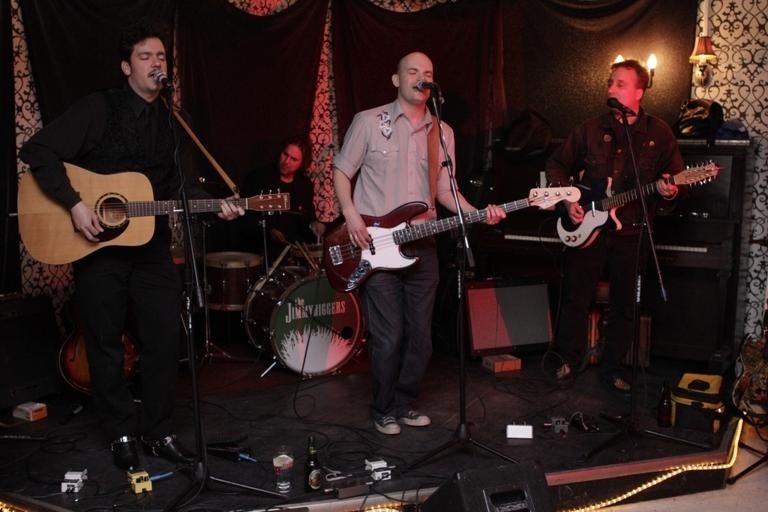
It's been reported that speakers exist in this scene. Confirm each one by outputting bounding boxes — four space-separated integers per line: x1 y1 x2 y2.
466 277 554 360
0 294 62 412
418 461 555 512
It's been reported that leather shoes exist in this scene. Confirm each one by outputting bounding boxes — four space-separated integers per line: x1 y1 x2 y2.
110 435 139 473
140 435 194 462
552 364 576 391
598 373 632 392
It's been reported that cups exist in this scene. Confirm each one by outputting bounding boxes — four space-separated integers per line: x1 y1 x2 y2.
273 454 294 494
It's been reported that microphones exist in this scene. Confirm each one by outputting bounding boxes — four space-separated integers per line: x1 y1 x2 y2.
607 97 636 115
153 69 173 91
417 80 436 90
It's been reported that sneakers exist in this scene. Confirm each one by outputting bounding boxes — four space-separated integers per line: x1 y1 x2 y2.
373 415 401 436
401 410 434 426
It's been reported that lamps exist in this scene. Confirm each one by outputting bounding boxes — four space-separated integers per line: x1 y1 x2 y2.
613 52 659 89
689 36 717 87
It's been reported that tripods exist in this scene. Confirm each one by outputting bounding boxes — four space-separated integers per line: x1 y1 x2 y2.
163 93 289 512
401 96 521 473
582 115 715 460
178 221 243 365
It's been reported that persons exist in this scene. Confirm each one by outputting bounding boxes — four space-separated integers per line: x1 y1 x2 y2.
18 26 248 467
242 140 335 240
335 52 506 436
545 60 688 387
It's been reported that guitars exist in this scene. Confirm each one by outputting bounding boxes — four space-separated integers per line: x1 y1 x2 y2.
322 183 582 294
17 162 292 265
556 160 720 249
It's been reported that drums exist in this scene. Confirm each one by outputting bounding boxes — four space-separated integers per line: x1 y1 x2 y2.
202 250 264 311
58 323 138 394
295 243 323 266
242 266 362 380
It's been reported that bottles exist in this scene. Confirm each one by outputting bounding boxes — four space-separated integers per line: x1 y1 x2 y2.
303 434 322 493
